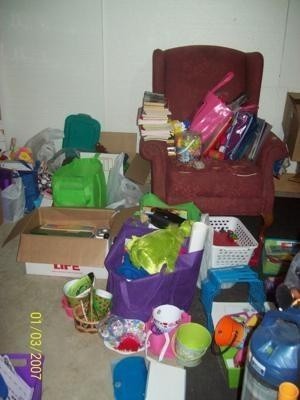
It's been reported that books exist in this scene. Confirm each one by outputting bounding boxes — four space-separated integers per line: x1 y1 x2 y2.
136 90 173 142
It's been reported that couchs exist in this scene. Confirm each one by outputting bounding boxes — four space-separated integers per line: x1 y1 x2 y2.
138 45 291 229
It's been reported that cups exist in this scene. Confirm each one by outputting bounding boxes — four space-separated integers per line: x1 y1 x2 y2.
94 289 113 320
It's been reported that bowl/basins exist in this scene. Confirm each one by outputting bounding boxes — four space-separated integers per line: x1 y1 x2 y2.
152 304 183 333
63 280 91 309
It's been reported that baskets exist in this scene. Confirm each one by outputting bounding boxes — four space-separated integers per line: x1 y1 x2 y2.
206 216 258 289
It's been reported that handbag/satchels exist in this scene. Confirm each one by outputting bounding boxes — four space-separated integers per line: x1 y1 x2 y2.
190 71 257 144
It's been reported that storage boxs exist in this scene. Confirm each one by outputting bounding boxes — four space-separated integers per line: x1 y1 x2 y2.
261 237 300 276
1 206 139 280
272 92 300 199
79 131 152 193
210 301 277 389
